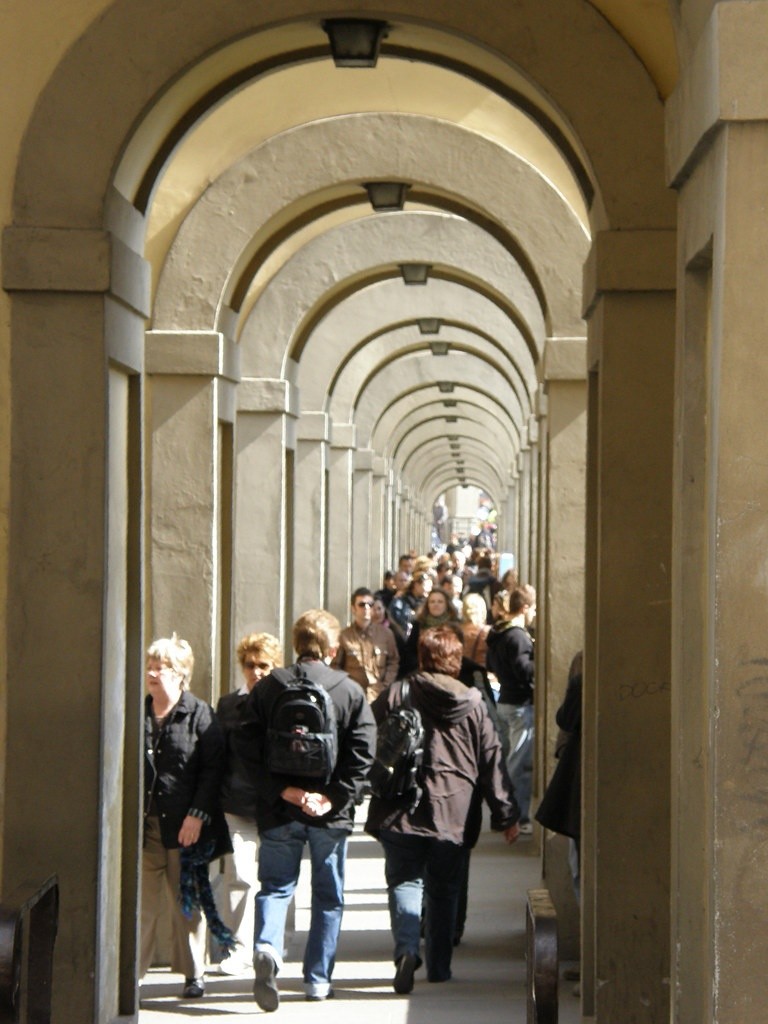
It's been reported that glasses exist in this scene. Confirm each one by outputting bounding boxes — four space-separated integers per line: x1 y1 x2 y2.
245 662 269 671
355 601 374 609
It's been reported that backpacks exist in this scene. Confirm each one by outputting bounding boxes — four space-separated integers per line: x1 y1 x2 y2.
366 674 424 804
265 665 349 785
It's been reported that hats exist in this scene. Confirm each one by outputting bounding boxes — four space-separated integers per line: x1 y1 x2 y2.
412 556 437 574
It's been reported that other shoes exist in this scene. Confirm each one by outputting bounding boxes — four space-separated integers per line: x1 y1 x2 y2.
304 982 334 1002
428 971 452 982
252 951 279 1013
521 823 533 834
393 951 416 994
183 974 205 998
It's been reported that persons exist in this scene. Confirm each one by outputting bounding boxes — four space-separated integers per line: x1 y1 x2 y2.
232 609 377 1012
330 522 584 836
144 626 584 999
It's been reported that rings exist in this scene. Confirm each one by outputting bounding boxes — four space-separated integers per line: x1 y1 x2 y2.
190 833 194 837
